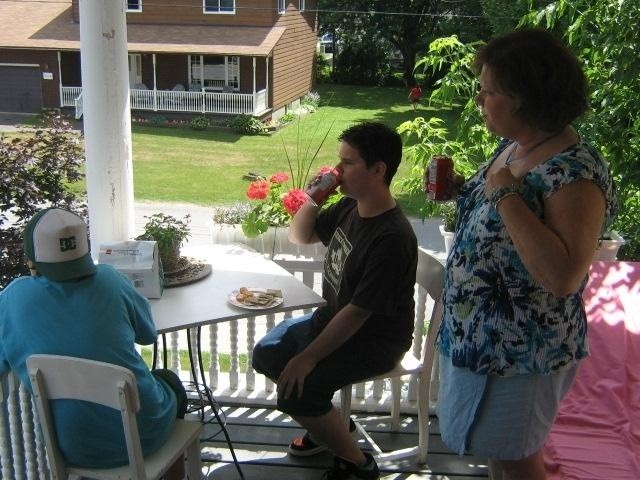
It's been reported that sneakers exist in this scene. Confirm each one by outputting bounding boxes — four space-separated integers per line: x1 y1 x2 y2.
318 452 379 480
288 417 358 457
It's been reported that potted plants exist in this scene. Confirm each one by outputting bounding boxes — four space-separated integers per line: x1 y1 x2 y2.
439 203 461 257
593 224 623 262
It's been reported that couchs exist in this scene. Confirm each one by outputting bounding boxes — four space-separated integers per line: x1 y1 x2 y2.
531 262 640 479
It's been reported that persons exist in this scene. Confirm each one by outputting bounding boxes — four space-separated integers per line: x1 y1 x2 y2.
0 205 190 478
248 120 419 478
418 26 617 478
408 84 423 112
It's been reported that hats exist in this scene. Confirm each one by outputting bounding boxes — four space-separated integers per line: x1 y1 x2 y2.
23 207 97 282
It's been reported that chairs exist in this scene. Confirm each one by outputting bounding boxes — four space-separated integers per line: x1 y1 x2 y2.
336 247 450 471
26 352 204 479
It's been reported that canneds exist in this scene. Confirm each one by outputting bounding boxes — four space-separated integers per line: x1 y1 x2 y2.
306 165 342 207
428 156 454 201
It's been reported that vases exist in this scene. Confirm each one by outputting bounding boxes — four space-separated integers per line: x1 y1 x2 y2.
158 244 182 270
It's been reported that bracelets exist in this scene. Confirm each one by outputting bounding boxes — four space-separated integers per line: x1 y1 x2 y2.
488 184 523 208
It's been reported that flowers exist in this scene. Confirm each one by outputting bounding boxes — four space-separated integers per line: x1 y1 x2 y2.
138 214 190 253
243 167 335 216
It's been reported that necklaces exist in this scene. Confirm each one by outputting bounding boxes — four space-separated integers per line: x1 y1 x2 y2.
499 122 571 173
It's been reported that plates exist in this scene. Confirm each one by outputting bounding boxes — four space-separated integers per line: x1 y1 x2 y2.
227 287 284 310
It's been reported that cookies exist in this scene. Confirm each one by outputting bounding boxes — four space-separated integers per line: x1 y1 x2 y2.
236 287 282 308
